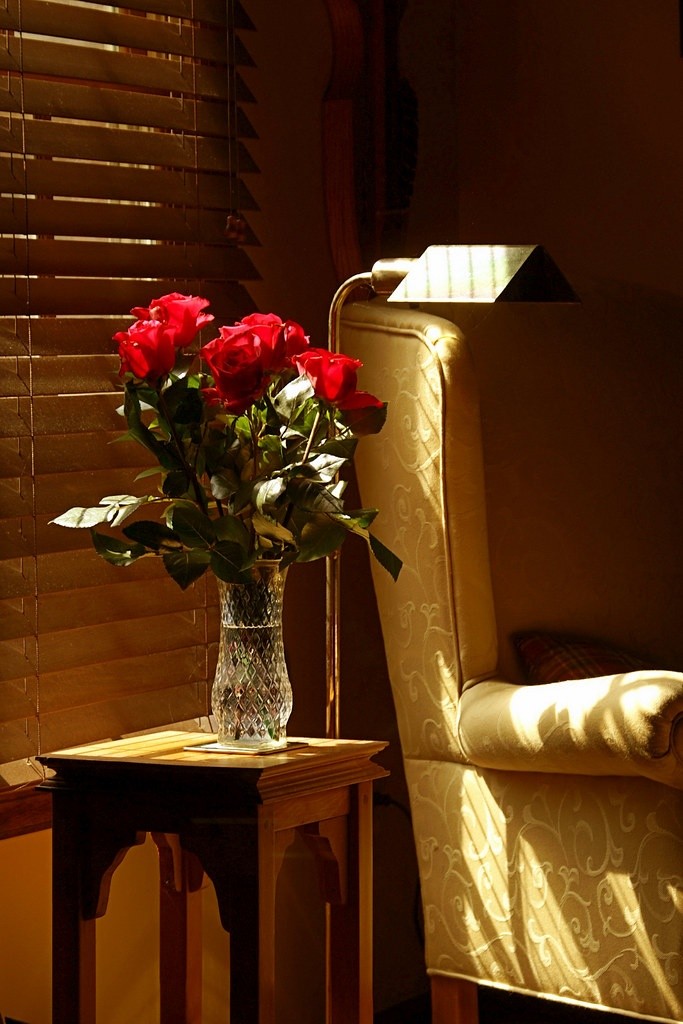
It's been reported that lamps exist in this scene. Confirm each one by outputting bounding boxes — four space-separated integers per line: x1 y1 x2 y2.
319 244 576 1024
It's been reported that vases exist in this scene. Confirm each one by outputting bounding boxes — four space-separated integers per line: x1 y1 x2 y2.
212 560 292 756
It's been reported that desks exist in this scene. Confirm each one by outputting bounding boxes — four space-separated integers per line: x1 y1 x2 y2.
35 730 390 1024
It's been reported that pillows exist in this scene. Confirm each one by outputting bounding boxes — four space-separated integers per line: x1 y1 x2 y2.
512 632 635 685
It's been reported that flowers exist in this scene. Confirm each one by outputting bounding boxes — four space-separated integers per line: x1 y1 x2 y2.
48 293 404 740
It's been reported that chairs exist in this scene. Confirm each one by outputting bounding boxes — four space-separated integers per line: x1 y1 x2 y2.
340 299 683 1024
